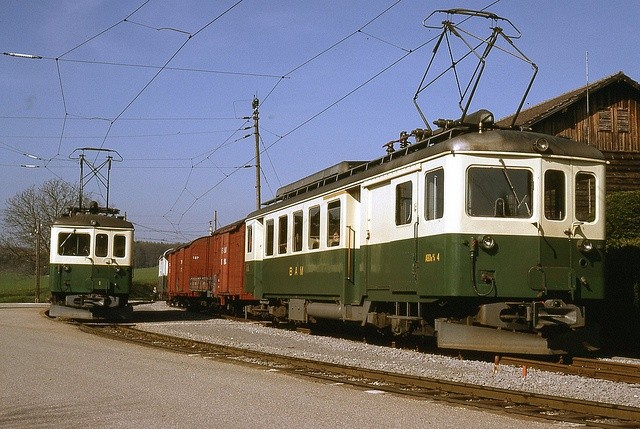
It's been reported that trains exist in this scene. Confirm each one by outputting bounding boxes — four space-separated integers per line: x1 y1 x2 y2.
46 202 134 323
158 109 609 366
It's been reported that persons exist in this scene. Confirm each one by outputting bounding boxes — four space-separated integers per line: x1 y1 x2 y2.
151 287 158 302
332 229 340 242
